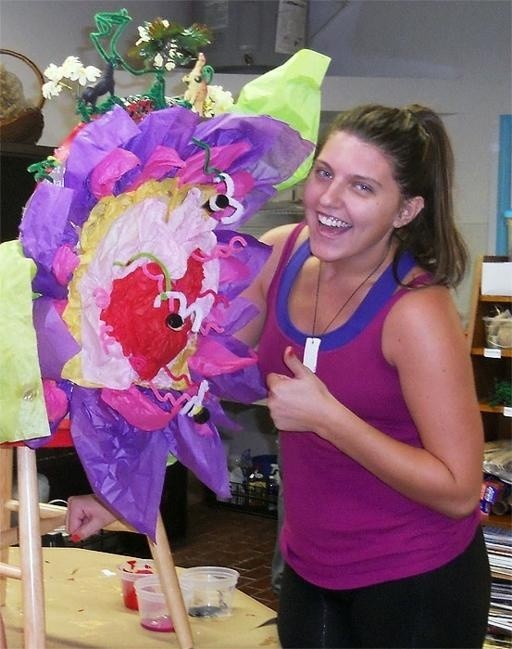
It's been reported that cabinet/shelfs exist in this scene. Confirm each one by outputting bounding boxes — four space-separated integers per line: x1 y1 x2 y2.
465 253 512 528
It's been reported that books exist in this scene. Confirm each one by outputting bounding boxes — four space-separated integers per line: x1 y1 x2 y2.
483 526 512 631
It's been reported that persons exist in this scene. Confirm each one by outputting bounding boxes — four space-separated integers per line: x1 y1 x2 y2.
67 103 490 648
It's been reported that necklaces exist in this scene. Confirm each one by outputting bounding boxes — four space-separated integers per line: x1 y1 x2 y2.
264 260 385 373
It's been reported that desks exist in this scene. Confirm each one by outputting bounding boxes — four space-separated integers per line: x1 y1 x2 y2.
1 547 291 648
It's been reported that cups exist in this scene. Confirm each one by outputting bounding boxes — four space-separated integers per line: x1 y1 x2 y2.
117 561 241 633
